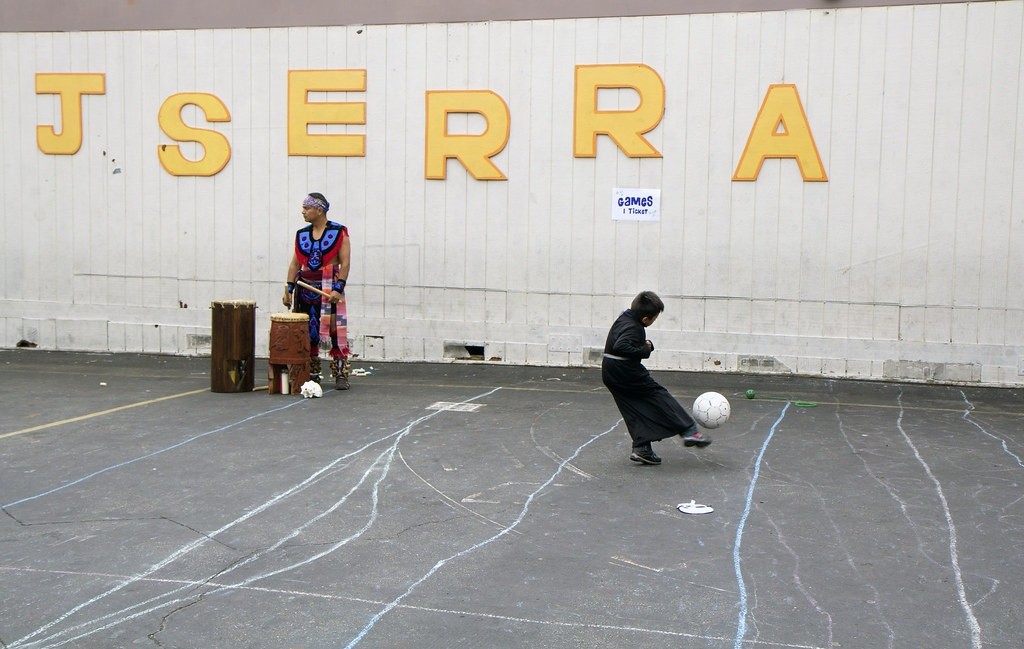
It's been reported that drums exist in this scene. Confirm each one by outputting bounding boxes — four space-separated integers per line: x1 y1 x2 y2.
210 298 258 394
268 311 311 396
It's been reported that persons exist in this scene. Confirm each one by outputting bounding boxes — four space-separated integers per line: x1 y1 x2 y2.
282 192 352 391
601 291 712 464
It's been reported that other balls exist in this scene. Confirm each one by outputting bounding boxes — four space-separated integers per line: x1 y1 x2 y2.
744 388 756 400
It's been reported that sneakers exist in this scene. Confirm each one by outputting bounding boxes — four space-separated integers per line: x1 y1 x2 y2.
684 433 711 448
629 448 661 465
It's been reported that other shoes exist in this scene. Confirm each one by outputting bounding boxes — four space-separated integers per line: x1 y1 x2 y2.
336 377 350 390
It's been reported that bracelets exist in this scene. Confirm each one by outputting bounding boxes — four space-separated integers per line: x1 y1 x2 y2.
332 279 346 294
286 282 295 294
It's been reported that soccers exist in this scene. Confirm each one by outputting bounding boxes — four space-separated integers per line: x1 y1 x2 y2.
691 392 731 429
